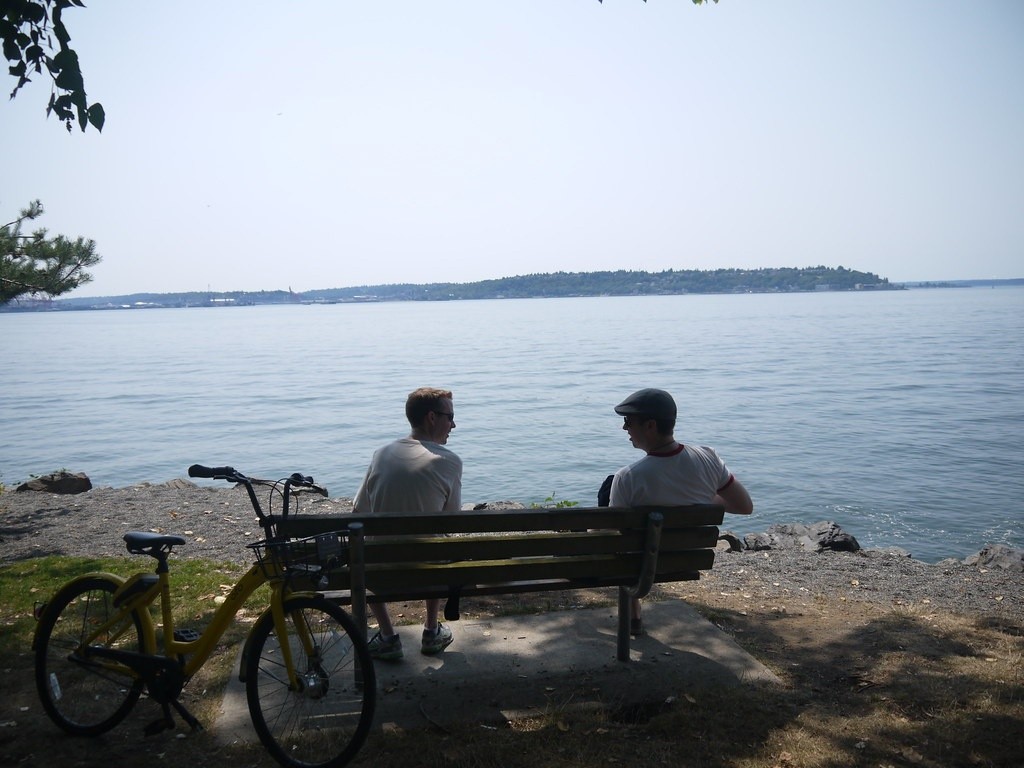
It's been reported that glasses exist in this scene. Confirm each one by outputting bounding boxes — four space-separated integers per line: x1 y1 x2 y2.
425 411 454 422
623 417 639 428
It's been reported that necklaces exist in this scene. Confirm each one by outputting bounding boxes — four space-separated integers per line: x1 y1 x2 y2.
653 440 676 451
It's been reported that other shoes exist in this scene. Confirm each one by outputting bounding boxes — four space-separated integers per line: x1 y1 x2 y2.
630 617 643 636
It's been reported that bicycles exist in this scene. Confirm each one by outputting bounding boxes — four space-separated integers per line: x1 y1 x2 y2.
31 466 380 767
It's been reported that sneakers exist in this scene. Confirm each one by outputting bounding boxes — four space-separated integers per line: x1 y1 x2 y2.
421 622 452 656
367 633 404 660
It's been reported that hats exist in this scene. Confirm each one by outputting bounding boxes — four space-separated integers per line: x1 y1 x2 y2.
614 388 677 416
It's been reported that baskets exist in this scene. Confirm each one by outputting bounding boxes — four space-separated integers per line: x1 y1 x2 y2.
247 529 352 583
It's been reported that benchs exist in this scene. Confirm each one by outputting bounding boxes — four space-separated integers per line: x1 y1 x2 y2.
259 502 726 687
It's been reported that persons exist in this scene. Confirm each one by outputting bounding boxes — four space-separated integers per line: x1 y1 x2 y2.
600 386 754 518
350 384 466 659
